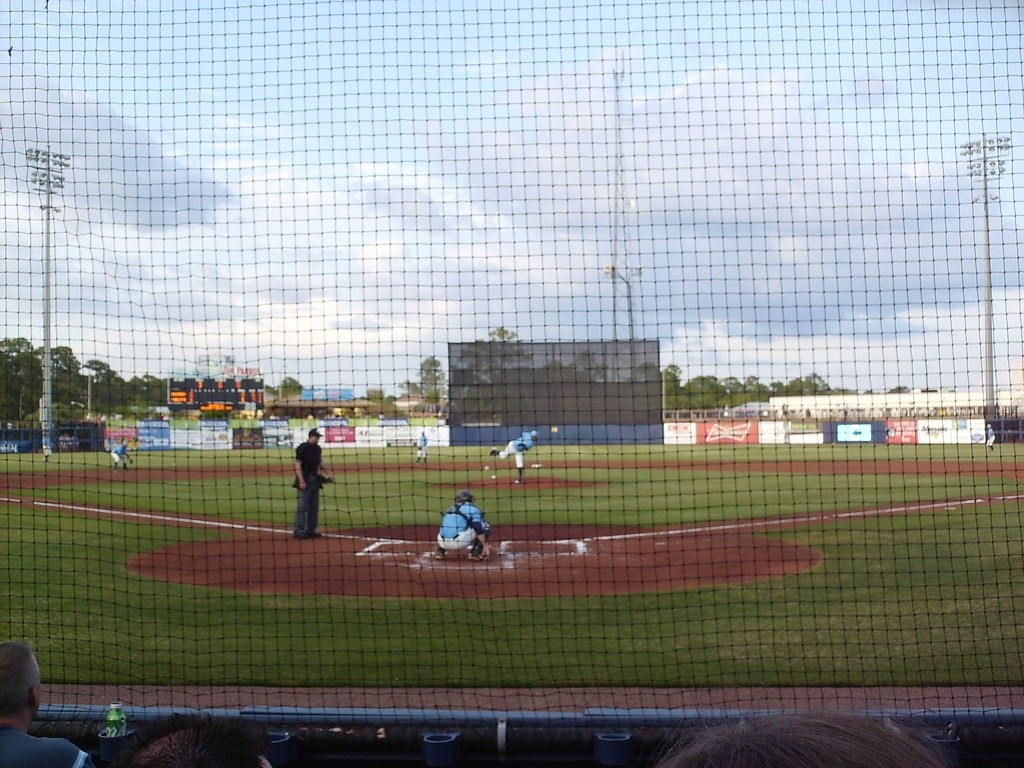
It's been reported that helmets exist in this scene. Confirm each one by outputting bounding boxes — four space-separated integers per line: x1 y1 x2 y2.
456 491 473 502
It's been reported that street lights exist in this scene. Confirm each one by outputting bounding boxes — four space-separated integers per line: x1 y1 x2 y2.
604 266 641 382
25 147 70 457
959 133 1010 421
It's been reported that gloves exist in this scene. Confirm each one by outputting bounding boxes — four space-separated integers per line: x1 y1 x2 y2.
485 530 491 542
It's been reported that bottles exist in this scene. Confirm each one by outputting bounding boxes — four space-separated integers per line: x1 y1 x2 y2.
106 702 126 737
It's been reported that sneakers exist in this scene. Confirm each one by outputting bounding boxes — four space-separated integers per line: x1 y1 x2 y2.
489 448 498 456
515 477 524 483
468 549 484 560
424 461 426 464
114 466 118 470
123 466 128 470
416 460 420 463
435 548 447 559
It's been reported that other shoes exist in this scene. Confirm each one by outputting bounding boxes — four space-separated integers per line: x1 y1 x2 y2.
294 531 324 539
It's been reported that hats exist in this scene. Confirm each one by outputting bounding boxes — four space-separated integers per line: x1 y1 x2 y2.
123 441 127 445
531 431 539 436
310 429 323 437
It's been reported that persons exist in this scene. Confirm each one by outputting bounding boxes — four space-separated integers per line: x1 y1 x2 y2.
434 491 490 561
1 639 1024 767
292 429 329 538
986 423 995 454
110 440 134 470
42 432 52 462
416 433 427 463
491 431 539 484
